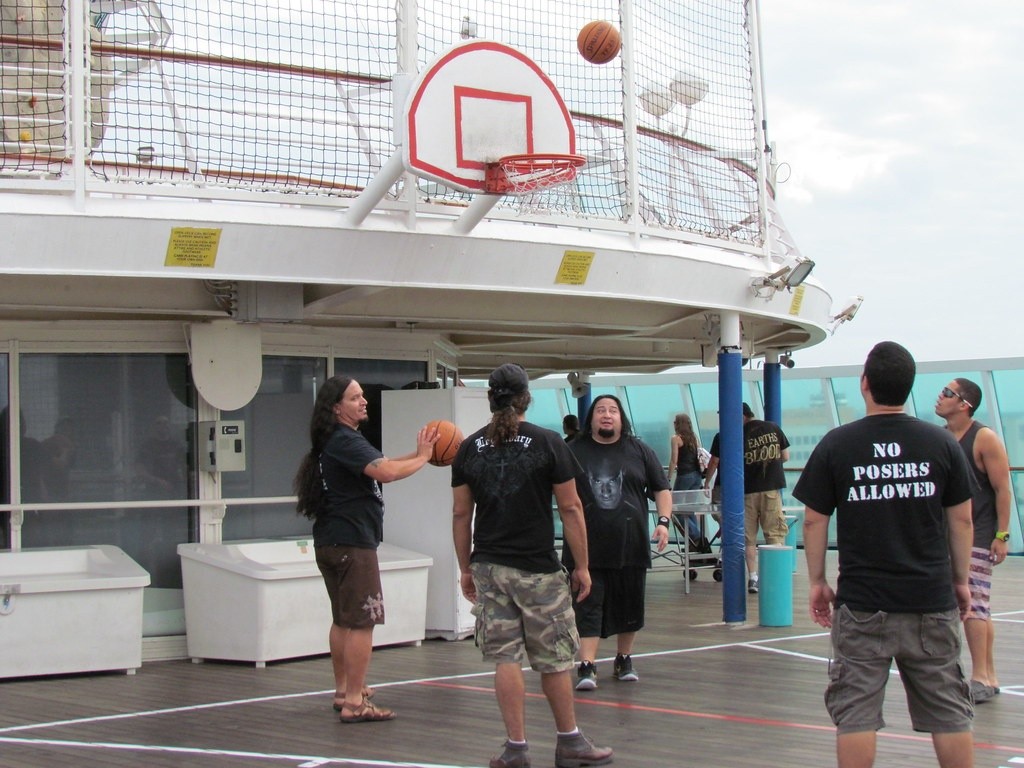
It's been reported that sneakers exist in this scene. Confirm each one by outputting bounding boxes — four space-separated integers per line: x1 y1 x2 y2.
575 660 597 690
613 652 638 680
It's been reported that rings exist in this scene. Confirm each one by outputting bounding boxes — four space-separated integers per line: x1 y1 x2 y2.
815 610 818 612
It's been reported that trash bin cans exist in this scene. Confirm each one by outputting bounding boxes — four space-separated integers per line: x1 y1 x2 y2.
755 544 795 628
783 515 798 574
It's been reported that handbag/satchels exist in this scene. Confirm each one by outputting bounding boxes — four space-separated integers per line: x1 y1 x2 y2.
696 447 713 479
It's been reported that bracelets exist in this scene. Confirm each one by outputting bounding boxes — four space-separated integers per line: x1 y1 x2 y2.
658 515 670 528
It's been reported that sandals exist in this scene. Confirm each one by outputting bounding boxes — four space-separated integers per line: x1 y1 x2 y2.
341 697 396 723
334 685 376 710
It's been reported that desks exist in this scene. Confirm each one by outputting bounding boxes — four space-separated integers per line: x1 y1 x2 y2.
646 506 806 594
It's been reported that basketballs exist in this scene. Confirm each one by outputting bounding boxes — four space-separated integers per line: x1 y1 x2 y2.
421 420 464 467
577 21 621 65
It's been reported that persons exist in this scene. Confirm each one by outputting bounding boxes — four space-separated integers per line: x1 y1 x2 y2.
291 375 441 722
791 340 975 768
557 395 673 691
561 413 581 442
450 361 613 768
704 409 721 522
0 408 186 588
934 377 1013 701
666 412 707 552
743 403 790 593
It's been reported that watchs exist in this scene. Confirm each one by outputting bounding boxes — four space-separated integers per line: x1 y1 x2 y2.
996 532 1010 542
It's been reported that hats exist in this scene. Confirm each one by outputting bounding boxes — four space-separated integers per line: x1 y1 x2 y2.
489 363 529 399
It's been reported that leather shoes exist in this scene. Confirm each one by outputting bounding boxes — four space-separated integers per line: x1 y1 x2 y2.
555 731 613 768
489 741 531 768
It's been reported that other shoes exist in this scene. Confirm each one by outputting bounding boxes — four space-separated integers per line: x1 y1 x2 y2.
990 686 1000 693
748 578 759 592
681 540 700 552
968 679 994 702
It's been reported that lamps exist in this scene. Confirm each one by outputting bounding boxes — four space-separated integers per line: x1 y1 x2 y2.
760 254 817 293
826 297 864 335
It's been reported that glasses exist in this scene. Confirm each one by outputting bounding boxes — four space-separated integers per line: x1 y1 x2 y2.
943 387 974 408
